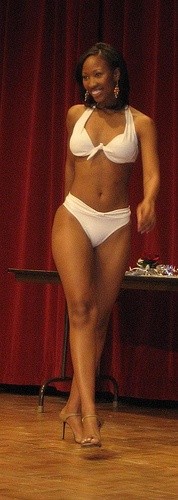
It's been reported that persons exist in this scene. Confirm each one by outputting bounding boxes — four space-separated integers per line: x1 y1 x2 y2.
49 40 160 447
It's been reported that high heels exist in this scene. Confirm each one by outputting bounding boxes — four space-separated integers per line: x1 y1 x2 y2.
58 408 85 444
79 411 105 448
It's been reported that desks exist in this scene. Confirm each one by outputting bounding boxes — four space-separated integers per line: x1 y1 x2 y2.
9 266 177 412
